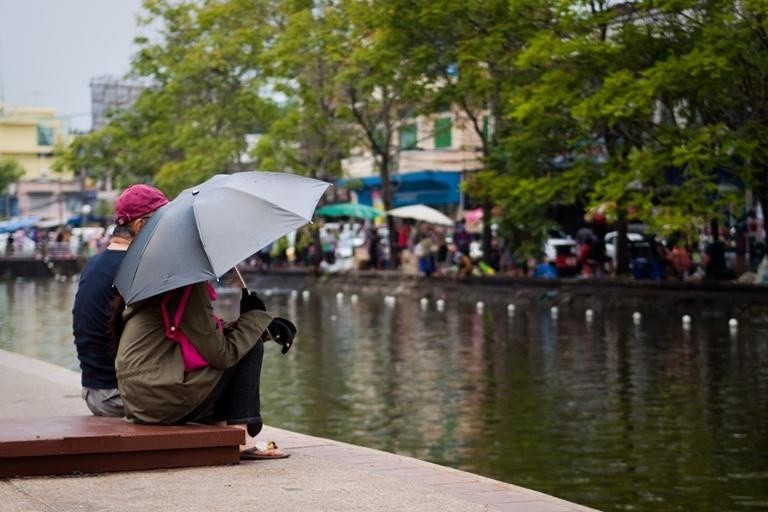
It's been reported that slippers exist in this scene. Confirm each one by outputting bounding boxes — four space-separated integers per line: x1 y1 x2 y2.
240 442 293 459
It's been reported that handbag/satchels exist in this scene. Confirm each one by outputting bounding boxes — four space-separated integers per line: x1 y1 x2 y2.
163 281 225 373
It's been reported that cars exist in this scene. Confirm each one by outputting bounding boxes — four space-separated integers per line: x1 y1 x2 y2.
320 222 391 270
546 226 645 272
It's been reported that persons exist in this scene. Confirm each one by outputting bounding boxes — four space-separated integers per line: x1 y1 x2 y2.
492 230 557 282
56 230 63 242
73 186 172 417
6 233 14 255
306 242 316 265
260 241 272 268
575 228 596 277
277 235 288 261
397 218 483 278
114 278 297 459
366 229 381 270
77 234 84 254
644 231 767 284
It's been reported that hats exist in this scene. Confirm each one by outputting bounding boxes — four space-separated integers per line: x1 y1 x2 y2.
113 183 170 222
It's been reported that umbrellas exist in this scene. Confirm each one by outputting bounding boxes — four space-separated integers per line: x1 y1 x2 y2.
314 202 383 219
386 204 455 227
112 172 333 309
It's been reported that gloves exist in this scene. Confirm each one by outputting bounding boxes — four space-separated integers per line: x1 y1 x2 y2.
266 317 298 353
237 289 266 314
